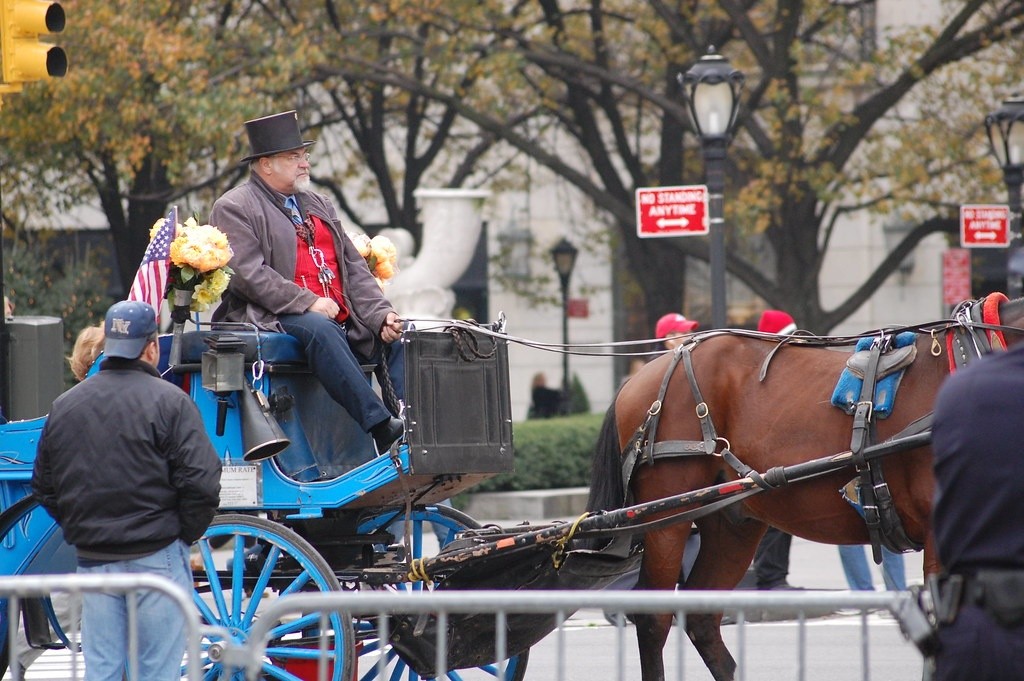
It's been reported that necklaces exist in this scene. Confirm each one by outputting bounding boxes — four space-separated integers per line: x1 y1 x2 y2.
292 213 336 298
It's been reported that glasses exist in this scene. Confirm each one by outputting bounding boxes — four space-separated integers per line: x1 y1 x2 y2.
270 153 311 161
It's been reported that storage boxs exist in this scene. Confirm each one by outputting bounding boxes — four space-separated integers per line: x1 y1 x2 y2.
267 636 363 681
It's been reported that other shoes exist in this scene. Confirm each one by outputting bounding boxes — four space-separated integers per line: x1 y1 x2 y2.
837 605 893 619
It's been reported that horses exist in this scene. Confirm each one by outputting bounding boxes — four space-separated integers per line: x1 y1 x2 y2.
578 295 1024 681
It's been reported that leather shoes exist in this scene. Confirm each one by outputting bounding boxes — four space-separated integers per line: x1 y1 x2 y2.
372 416 405 455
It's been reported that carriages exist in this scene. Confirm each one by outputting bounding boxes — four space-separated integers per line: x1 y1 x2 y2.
0 293 1023 681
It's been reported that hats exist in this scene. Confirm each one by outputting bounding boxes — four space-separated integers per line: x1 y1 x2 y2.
758 309 797 335
239 110 316 162
655 313 699 337
105 300 156 358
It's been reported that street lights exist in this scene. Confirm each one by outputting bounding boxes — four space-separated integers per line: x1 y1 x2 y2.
984 93 1024 300
676 44 745 327
549 238 580 414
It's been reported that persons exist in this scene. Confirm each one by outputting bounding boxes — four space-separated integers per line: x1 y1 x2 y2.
206 108 405 457
30 300 223 681
651 307 907 591
530 370 560 417
929 334 1024 681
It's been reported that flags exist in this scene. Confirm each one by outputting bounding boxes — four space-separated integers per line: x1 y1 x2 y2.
123 203 177 337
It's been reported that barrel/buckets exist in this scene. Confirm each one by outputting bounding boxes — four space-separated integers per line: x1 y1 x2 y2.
256 637 364 681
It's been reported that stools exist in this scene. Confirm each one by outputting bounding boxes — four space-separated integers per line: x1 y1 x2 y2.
181 331 378 479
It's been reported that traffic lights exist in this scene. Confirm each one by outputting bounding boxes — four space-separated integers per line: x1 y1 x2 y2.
0 0 67 95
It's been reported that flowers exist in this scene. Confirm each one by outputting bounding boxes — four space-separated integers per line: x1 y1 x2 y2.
344 222 396 295
142 213 235 311
